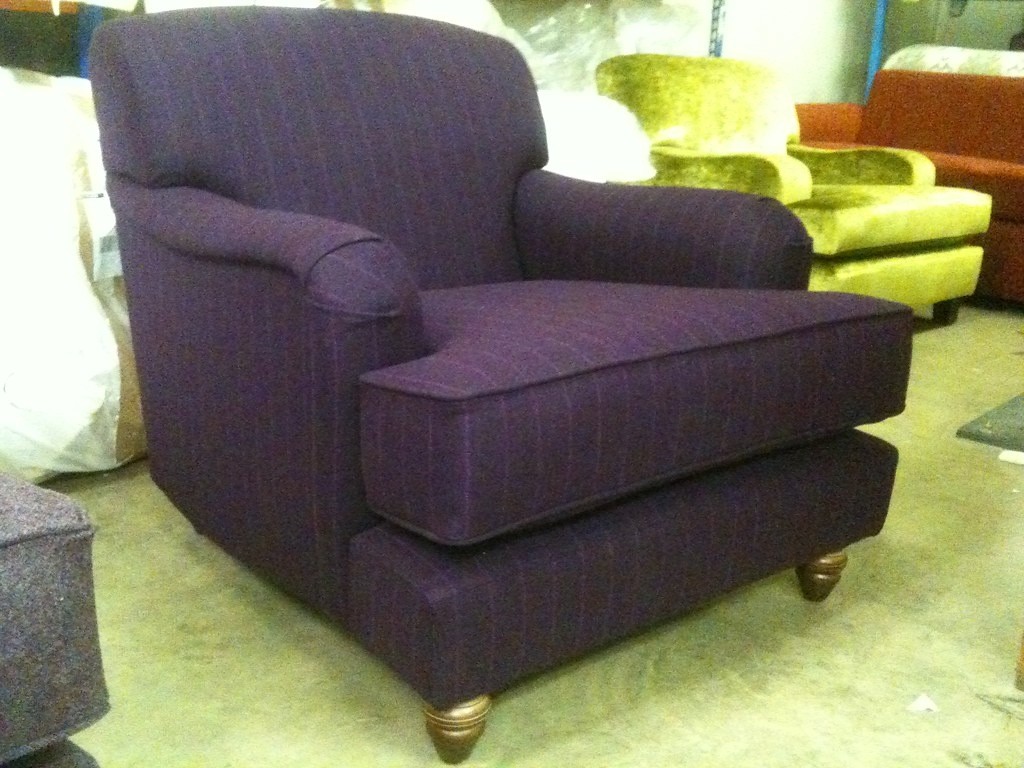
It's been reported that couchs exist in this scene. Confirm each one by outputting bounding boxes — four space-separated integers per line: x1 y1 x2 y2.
595 44 1024 324
89 6 913 764
0 472 111 768
